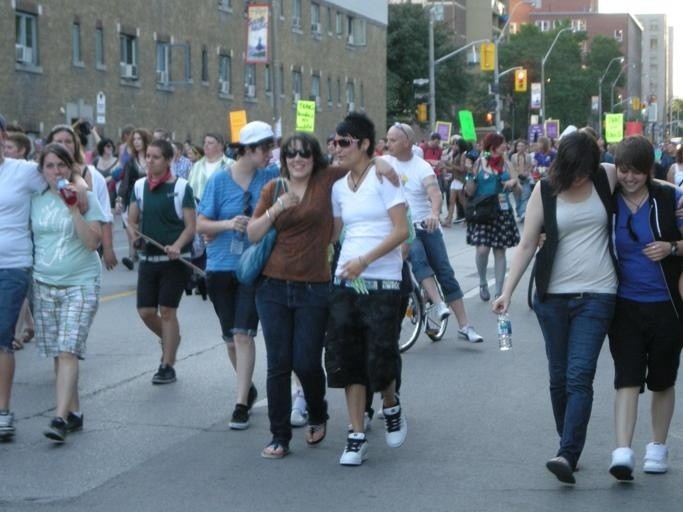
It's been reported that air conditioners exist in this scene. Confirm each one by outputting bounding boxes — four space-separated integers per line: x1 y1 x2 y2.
217 78 367 115
118 61 139 80
292 15 304 29
154 69 170 86
311 22 321 34
14 43 34 64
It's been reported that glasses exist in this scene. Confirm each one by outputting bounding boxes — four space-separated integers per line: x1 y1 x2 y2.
627 215 637 240
334 138 360 146
244 192 252 215
287 148 311 158
395 122 408 138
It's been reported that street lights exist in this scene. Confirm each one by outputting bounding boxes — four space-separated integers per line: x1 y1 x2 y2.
494 1 533 130
597 56 635 139
428 15 490 134
540 28 572 136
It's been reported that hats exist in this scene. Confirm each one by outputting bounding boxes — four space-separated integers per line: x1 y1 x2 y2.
228 121 274 146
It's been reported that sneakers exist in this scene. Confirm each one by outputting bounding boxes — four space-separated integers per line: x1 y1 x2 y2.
339 395 407 465
229 383 257 429
45 417 65 440
608 447 635 480
437 302 449 320
480 280 489 300
0 414 14 437
643 442 668 473
152 364 175 384
290 396 309 427
161 335 180 361
67 412 82 432
458 324 483 342
123 258 133 269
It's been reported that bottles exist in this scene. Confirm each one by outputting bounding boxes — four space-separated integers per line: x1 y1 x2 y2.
55 174 76 205
496 305 512 350
231 231 244 255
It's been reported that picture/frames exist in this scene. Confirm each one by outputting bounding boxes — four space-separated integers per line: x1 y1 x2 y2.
434 120 452 144
544 119 560 140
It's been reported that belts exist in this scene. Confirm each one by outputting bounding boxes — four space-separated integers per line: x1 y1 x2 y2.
335 277 400 290
140 251 191 261
549 293 584 299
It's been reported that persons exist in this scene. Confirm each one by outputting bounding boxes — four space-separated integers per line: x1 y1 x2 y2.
1 111 683 484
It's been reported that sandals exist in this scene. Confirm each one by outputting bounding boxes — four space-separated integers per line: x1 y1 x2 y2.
12 328 34 349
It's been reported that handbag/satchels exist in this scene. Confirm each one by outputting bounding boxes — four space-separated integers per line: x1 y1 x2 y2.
465 192 497 222
235 226 277 286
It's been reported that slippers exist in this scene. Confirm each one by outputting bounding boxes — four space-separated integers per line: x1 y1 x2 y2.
306 422 326 444
546 458 575 483
261 442 288 458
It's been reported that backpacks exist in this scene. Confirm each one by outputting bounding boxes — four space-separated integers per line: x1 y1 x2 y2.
133 176 205 259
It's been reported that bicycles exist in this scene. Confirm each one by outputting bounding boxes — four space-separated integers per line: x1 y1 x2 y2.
398 261 448 353
